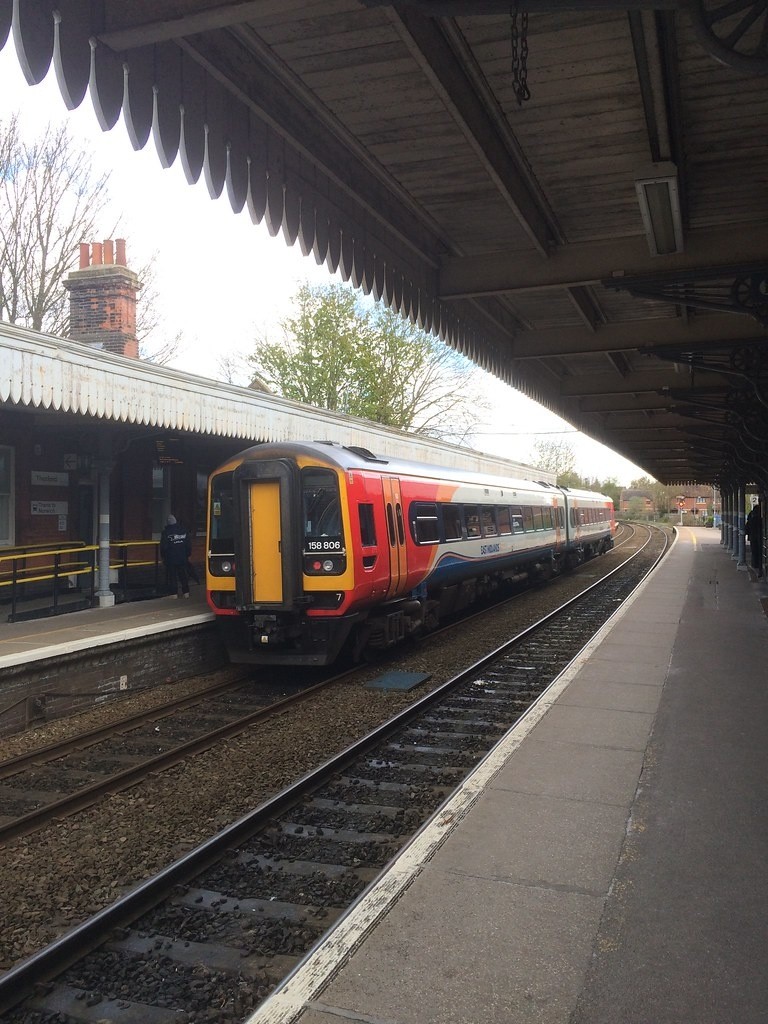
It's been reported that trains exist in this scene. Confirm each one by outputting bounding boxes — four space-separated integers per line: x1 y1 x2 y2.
206 438 617 668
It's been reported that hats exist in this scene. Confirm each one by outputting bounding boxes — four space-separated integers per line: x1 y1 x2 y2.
168 515 176 525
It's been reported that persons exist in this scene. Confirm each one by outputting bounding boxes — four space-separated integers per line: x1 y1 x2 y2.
161 515 193 600
744 504 761 569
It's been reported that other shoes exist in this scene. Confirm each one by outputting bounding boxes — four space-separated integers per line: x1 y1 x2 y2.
167 595 178 599
183 593 189 599
